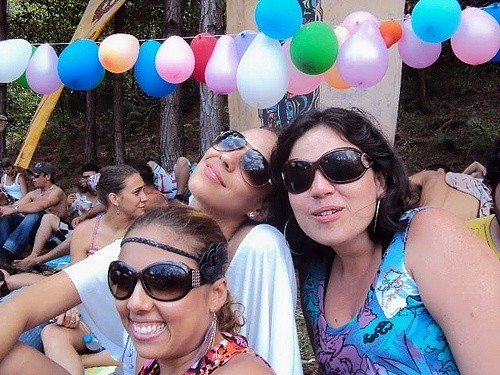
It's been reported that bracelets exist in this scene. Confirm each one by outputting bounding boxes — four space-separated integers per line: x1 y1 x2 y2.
14 206 18 212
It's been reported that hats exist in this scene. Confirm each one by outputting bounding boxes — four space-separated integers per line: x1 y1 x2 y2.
26 162 55 176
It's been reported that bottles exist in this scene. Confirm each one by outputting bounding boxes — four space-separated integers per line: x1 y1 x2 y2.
82 333 105 352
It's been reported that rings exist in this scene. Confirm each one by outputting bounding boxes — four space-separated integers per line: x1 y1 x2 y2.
71 321 76 323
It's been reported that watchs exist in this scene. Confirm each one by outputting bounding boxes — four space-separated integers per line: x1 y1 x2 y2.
482 167 487 176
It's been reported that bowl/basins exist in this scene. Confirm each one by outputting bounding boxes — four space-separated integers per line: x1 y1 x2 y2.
81 201 92 209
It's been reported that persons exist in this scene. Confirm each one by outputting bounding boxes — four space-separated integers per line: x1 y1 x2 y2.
0 107 500 375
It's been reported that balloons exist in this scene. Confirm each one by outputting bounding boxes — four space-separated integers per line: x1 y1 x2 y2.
0 0 500 110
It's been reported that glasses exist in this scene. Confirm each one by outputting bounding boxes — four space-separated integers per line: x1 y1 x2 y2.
106 259 206 302
212 129 276 189
278 146 374 194
31 172 39 178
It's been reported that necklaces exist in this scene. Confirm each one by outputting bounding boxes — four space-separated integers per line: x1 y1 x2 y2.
40 185 55 196
